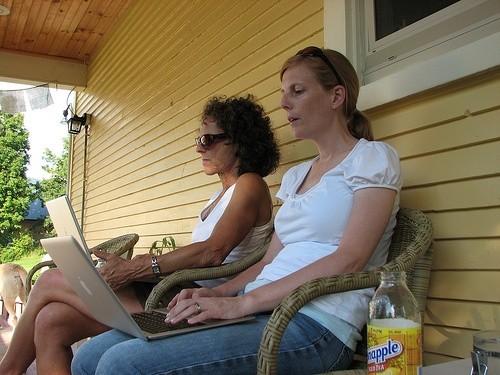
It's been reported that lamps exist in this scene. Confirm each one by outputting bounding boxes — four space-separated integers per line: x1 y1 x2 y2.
68 112 85 135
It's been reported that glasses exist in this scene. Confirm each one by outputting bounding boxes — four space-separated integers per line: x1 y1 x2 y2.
296 47 344 85
195 133 227 147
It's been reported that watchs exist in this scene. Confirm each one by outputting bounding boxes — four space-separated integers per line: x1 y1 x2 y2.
152 255 161 275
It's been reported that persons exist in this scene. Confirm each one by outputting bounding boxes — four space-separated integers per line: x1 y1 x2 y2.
0 93 282 375
71 46 403 375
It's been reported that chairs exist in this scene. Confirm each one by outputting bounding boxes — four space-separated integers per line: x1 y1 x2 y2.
145 197 437 375
25 231 141 306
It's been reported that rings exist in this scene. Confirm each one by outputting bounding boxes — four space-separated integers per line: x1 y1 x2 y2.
193 302 201 314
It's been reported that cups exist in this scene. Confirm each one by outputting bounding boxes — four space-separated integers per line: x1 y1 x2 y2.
469 330 500 375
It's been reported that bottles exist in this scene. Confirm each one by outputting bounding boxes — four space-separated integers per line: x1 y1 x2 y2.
366 270 422 375
